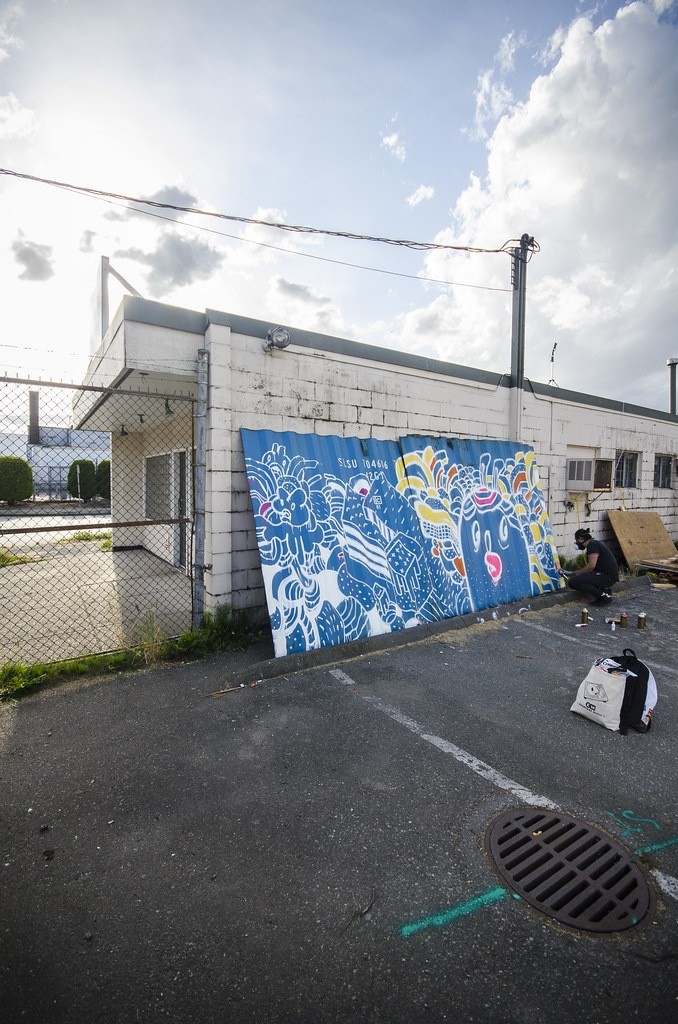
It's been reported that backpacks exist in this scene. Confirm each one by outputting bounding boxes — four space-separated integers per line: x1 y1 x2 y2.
611 648 658 733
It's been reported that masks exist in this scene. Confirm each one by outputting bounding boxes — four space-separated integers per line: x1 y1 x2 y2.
577 541 587 550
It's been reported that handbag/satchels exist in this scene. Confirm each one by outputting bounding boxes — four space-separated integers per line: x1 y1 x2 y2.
570 658 639 735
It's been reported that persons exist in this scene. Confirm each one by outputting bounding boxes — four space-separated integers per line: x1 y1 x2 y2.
558 528 618 606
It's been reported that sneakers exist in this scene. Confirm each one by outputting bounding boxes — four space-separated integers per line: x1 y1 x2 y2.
590 593 613 607
604 587 613 596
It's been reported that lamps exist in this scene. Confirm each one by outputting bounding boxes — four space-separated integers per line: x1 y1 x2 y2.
137 413 146 423
165 398 174 418
262 326 293 353
119 425 128 436
